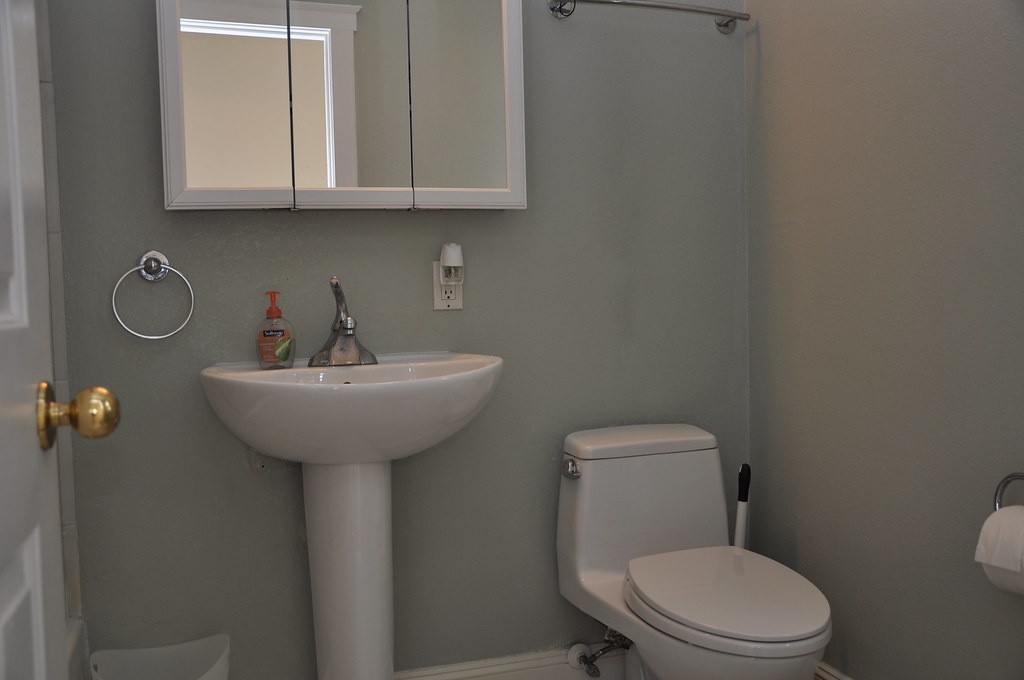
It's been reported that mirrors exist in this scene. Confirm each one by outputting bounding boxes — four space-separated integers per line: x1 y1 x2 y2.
155 0 529 211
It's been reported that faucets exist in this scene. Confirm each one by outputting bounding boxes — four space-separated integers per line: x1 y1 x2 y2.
307 273 379 368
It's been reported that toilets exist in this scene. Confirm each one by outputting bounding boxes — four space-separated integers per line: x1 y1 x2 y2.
554 420 834 680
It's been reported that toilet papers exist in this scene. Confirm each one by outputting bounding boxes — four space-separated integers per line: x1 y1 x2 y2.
973 503 1024 595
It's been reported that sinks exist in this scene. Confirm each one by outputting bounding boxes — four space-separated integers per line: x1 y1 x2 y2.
201 348 505 466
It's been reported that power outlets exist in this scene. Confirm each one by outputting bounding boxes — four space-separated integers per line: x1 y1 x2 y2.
432 262 463 310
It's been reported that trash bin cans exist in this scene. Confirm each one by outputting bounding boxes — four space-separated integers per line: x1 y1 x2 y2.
90 633 230 680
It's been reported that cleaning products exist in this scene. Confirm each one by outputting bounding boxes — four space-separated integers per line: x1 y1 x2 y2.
254 290 298 371
